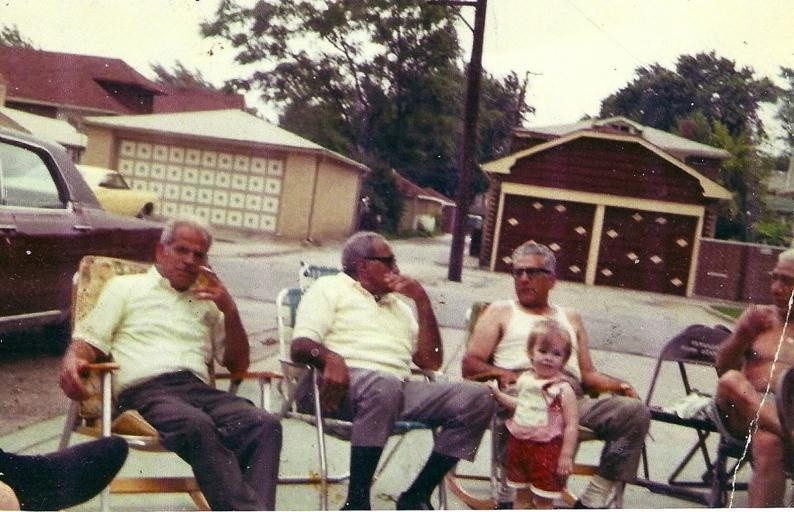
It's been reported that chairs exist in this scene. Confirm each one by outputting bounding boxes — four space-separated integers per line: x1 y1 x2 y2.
448 298 628 509
634 323 734 490
708 409 794 505
271 263 449 510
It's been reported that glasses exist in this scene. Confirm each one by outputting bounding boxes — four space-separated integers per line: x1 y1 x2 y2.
362 253 395 266
509 264 553 277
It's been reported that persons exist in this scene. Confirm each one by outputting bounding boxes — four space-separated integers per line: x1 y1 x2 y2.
289 230 494 510
486 319 583 508
460 240 651 510
1 435 129 512
716 247 794 507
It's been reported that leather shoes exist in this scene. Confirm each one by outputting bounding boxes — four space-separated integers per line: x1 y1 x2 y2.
30 434 128 512
395 488 436 510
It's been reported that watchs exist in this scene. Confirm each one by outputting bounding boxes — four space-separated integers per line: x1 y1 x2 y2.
308 345 323 360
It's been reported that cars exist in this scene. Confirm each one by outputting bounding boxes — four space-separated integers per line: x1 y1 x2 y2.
0 126 165 333
